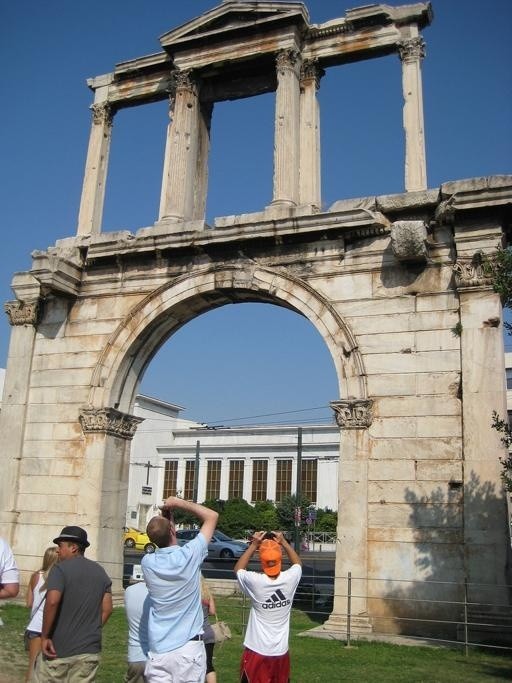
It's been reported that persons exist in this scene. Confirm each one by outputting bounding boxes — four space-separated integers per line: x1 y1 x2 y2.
234 530 304 682
141 495 221 683
0 530 22 630
23 546 62 682
26 526 113 683
198 570 219 682
123 579 151 682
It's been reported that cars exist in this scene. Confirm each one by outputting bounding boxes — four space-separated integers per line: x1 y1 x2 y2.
175 528 250 563
124 525 159 554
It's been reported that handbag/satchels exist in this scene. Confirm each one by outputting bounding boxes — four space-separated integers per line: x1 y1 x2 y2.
210 621 232 641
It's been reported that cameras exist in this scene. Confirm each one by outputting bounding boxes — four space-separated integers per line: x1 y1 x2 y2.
262 532 276 540
158 505 169 511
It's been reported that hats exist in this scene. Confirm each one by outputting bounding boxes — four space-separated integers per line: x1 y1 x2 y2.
53 526 91 546
260 540 282 577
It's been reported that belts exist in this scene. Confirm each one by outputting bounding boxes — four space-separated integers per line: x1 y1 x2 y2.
191 635 203 641
